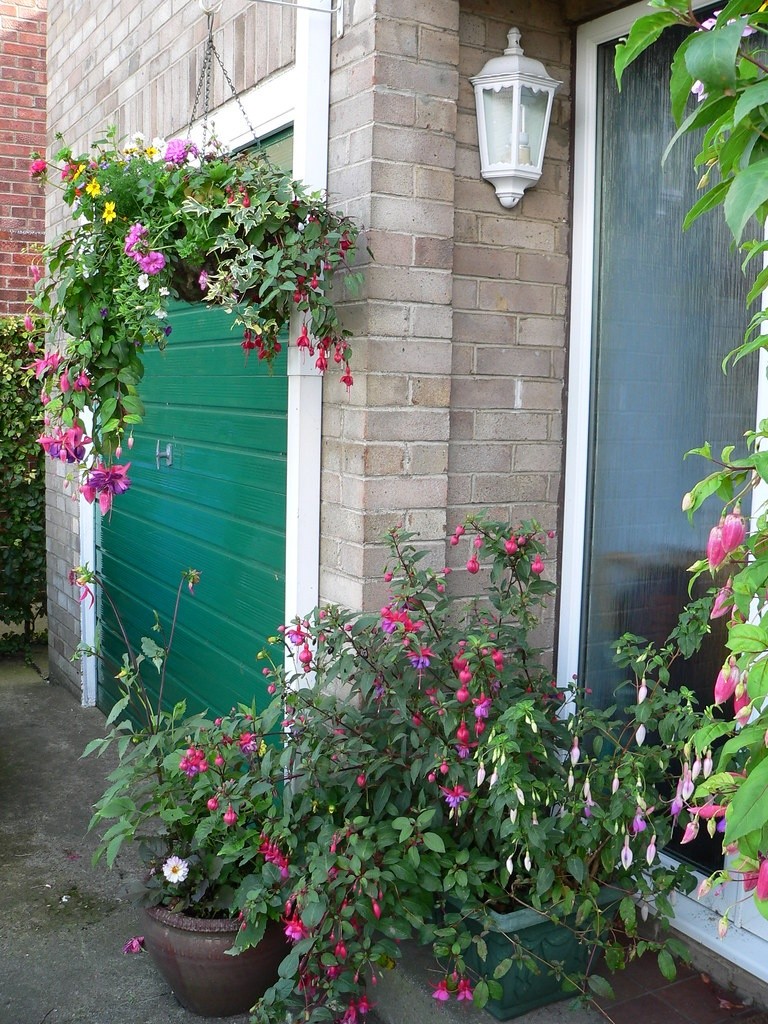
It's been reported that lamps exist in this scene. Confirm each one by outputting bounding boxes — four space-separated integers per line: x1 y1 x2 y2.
470 28 567 210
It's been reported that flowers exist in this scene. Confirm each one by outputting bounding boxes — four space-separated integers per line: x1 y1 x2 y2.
671 486 768 939
177 508 669 1024
15 123 371 516
63 561 298 921
682 6 761 104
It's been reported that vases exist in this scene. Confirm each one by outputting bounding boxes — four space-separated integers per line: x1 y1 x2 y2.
137 871 293 1018
165 260 237 303
429 862 639 1021
